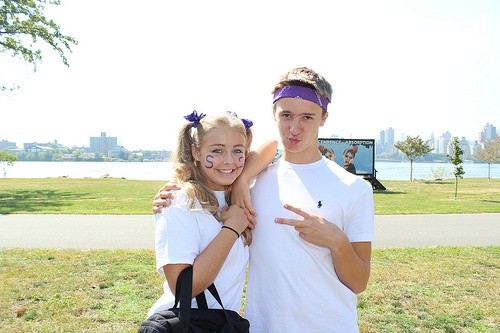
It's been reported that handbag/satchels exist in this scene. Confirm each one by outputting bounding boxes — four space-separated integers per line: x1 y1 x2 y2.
137 265 250 333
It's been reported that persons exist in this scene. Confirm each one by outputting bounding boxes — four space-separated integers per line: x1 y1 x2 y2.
147 110 279 317
153 66 373 333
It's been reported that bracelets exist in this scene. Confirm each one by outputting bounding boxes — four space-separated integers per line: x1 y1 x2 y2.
222 226 239 238
